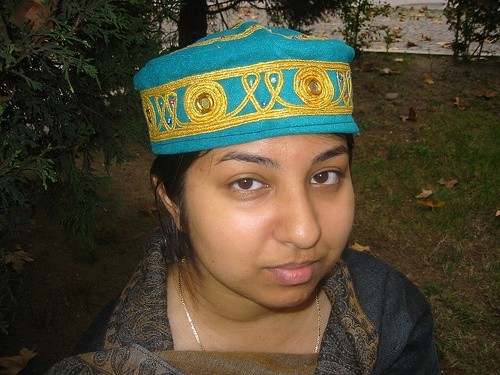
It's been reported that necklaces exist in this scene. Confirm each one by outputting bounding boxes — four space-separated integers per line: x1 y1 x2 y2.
176 254 321 356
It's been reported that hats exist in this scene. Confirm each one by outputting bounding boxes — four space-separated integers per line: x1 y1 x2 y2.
137 20 361 156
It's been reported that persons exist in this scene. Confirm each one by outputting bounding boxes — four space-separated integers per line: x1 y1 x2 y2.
51 21 443 375
